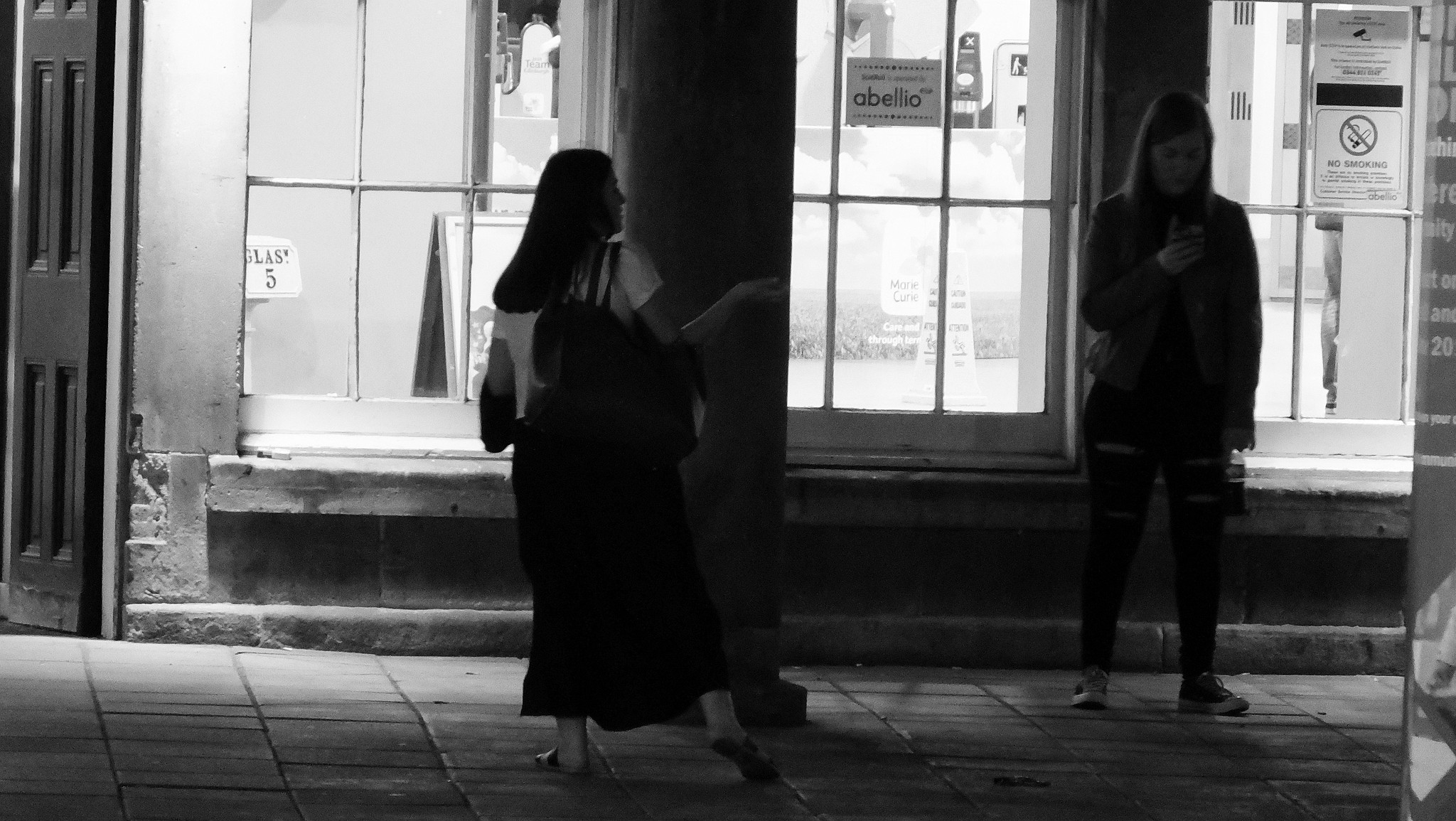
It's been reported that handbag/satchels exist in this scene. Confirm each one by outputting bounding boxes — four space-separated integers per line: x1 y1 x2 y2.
525 240 708 466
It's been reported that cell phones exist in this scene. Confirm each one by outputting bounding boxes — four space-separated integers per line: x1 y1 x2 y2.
1173 229 1202 252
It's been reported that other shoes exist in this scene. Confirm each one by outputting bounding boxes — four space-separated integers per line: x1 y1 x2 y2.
1070 669 1112 708
1177 670 1250 714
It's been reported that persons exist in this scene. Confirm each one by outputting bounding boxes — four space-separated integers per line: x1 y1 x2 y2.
478 149 791 782
1075 90 1264 714
1316 213 1345 414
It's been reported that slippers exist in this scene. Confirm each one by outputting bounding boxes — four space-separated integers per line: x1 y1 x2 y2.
535 744 590 774
711 734 780 783
